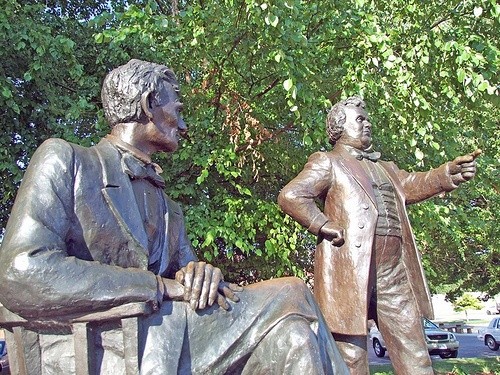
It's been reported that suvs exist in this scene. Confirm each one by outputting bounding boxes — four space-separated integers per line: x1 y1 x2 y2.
483 316 500 351
370 316 460 359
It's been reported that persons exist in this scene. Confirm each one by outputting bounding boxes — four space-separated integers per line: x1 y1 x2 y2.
277 97 484 375
0 58 352 375
